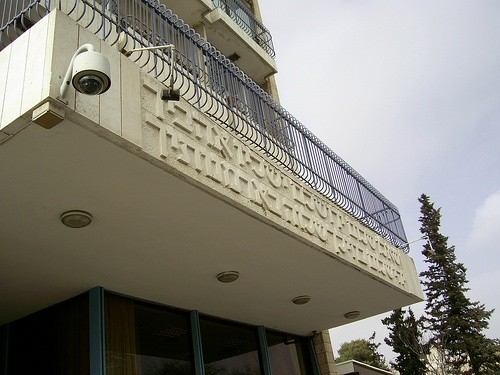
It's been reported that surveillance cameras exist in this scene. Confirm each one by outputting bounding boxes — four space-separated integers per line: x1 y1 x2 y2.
70 51 111 96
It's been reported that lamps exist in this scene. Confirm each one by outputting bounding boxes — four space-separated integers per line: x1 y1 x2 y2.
344 310 360 319
59 210 94 229
292 295 311 304
216 270 239 283
124 44 181 101
